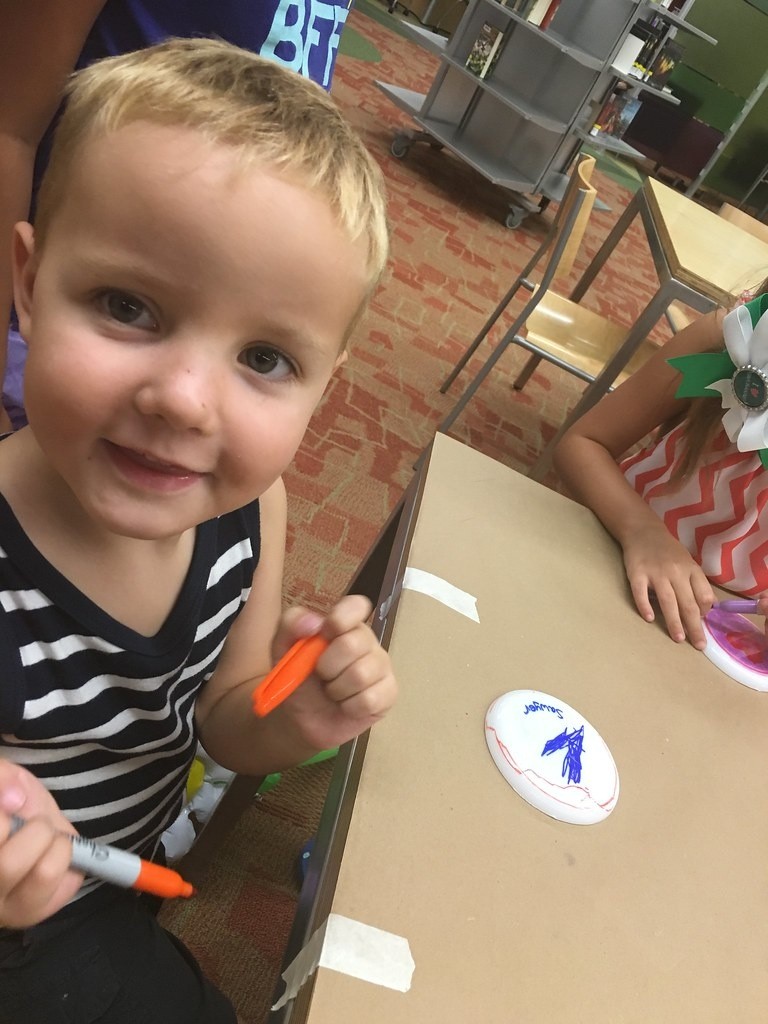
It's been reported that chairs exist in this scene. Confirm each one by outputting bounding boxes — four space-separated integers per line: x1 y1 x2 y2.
410 151 663 471
663 202 768 336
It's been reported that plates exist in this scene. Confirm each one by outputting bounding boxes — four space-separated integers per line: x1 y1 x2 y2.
700 612 768 692
486 690 619 826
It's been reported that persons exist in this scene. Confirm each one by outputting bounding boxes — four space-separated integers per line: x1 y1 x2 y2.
552 276 767 650
0 0 398 1024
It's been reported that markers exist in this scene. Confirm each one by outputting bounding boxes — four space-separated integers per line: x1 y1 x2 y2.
712 599 760 614
7 813 197 899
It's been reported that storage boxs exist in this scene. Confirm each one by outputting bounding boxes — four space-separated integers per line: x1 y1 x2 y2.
621 88 724 179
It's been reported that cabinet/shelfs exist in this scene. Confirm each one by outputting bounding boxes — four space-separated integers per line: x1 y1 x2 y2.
373 0 718 231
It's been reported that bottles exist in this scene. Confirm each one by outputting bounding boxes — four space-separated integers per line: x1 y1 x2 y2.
589 124 602 137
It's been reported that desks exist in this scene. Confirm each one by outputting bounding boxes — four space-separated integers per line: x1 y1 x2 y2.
512 176 768 486
169 432 768 1023
